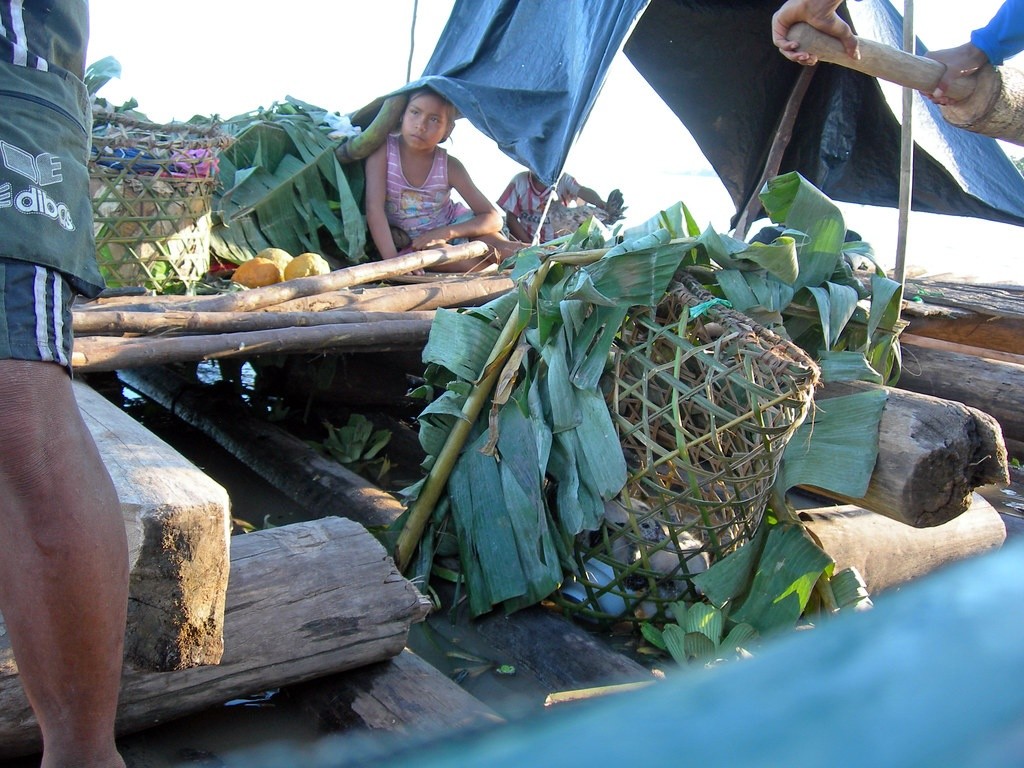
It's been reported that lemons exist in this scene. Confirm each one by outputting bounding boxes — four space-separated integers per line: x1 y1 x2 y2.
230 247 331 289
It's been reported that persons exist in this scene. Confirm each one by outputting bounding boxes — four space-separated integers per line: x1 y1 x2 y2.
496 170 619 243
0 0 129 768
365 87 503 277
771 0 1024 105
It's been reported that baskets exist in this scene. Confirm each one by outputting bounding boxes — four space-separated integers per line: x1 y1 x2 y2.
88 110 231 297
543 270 821 639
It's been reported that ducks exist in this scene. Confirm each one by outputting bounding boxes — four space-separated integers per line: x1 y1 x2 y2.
578 497 710 605
